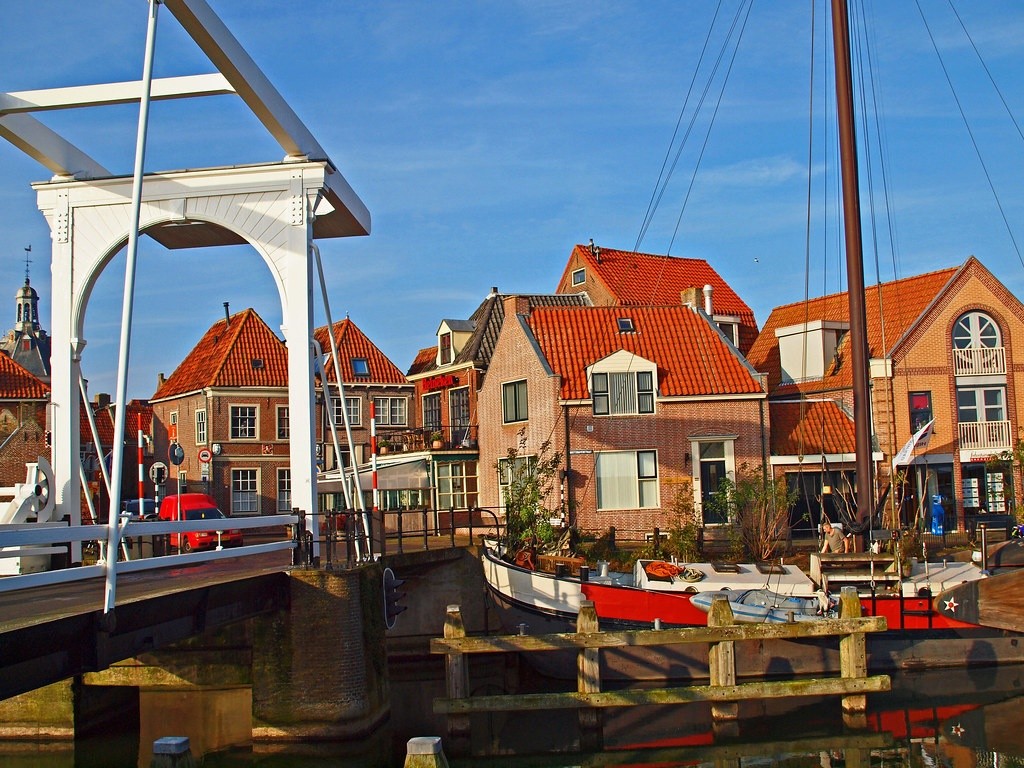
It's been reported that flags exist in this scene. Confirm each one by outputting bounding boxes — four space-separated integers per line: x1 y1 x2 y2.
886 417 934 476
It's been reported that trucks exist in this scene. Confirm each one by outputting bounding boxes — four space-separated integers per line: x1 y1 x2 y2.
159 493 243 554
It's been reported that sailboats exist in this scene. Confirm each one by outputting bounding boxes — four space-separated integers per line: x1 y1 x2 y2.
480 0 1024 681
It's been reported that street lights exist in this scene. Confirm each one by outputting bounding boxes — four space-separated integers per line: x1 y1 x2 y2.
89 403 116 482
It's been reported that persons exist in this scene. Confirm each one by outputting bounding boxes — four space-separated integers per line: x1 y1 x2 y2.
821 522 849 554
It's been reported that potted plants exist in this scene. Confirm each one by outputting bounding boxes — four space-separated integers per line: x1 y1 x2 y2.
378 439 390 455
969 539 982 562
902 559 914 578
430 430 444 448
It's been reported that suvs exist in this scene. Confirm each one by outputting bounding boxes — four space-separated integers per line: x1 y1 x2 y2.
121 498 161 521
